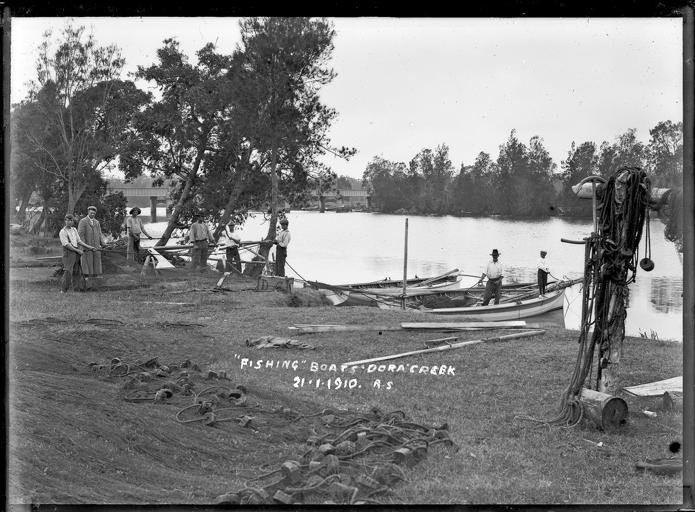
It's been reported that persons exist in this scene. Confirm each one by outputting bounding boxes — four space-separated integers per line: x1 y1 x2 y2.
126 206 153 264
537 250 550 298
57 213 96 293
221 221 243 273
189 211 217 273
77 206 108 291
274 219 292 277
478 249 504 306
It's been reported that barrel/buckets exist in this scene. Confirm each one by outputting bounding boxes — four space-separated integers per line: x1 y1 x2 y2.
580 387 628 434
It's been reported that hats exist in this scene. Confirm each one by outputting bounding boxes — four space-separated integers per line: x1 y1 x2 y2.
130 207 140 214
195 212 206 216
490 249 501 256
280 220 288 224
87 206 97 211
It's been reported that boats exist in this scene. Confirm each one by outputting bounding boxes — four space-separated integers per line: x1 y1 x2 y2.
317 264 567 323
